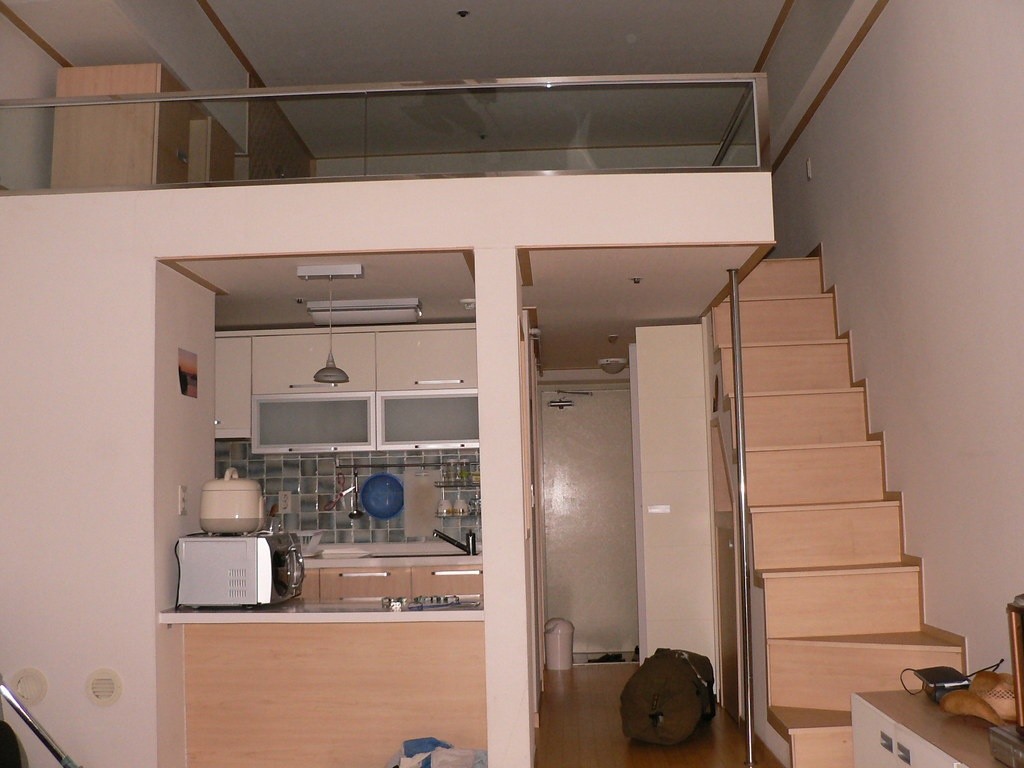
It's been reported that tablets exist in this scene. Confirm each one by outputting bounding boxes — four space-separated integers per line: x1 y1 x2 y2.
914 666 971 687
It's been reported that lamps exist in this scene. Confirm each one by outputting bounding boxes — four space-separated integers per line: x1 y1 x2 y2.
598 358 627 374
307 298 421 325
312 276 349 383
460 298 476 310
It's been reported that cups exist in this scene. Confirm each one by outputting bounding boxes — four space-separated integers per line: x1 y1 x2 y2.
446 458 457 482
459 459 470 482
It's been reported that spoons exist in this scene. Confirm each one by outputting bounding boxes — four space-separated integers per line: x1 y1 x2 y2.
348 474 364 520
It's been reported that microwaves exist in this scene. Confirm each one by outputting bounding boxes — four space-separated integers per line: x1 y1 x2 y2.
174 532 305 610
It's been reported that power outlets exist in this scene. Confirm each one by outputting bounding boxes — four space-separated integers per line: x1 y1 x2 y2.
178 485 188 516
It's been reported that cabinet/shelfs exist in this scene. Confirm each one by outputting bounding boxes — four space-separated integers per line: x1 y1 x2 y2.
215 322 480 544
188 114 238 182
295 565 483 606
51 64 189 189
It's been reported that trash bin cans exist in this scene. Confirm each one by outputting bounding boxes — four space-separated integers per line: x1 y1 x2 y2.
544 617 574 670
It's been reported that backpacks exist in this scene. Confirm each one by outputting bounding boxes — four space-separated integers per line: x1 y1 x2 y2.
619 648 715 745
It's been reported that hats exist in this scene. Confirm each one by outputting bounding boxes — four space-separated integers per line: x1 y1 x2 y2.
941 670 1020 729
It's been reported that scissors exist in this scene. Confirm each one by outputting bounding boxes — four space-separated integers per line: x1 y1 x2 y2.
324 472 355 511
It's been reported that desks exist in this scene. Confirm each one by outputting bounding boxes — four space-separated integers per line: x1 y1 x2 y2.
160 605 488 768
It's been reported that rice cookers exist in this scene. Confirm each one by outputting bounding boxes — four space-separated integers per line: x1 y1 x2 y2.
200 468 273 535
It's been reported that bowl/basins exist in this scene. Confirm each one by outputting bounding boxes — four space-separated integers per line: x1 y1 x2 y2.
437 500 469 516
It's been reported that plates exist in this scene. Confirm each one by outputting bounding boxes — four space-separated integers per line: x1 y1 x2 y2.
360 473 404 521
